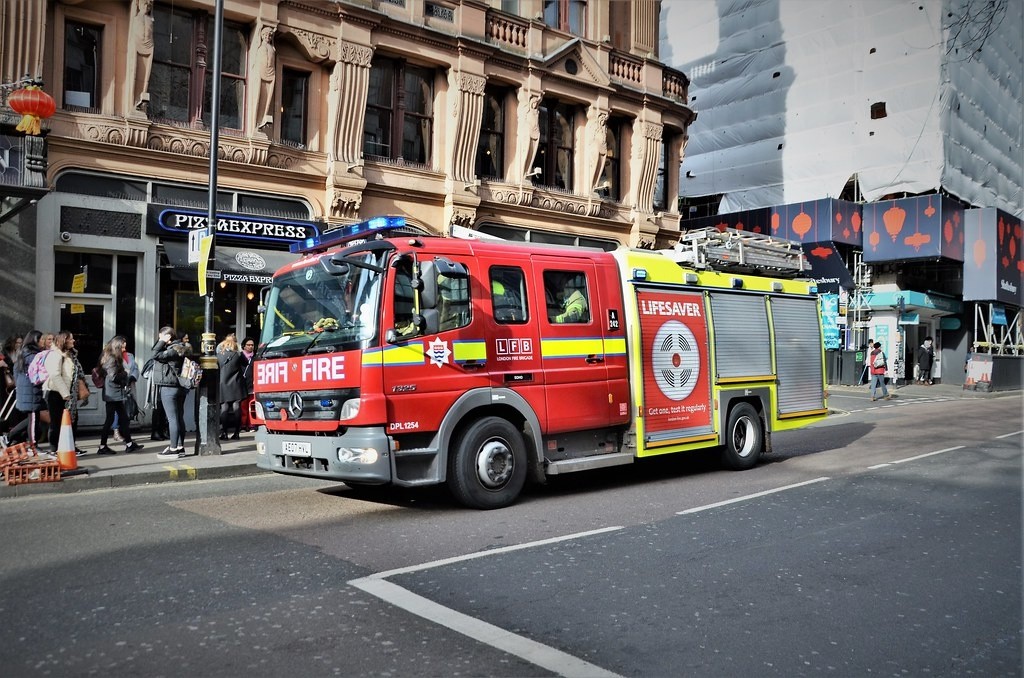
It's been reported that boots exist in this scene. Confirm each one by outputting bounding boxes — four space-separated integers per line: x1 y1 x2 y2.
151 413 165 441
160 415 169 439
114 428 124 441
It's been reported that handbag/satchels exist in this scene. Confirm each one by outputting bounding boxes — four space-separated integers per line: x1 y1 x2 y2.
873 352 884 368
77 379 90 401
913 365 920 380
124 393 146 421
4 368 14 387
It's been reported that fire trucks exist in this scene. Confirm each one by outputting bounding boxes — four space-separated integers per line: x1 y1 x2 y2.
248 214 831 511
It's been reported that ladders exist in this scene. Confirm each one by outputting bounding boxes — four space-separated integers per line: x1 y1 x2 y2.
680 227 812 271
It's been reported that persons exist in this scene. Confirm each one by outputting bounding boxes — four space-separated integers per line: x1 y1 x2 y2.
548 283 590 323
97 336 145 454
142 327 190 458
965 347 975 364
918 336 934 385
870 342 891 401
493 281 523 320
108 335 140 441
0 331 89 455
866 339 878 389
238 337 259 432
216 334 249 440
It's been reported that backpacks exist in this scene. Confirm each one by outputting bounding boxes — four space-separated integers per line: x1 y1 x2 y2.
177 356 203 389
92 364 105 388
28 350 54 386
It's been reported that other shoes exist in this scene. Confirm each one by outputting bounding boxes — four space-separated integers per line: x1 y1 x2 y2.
48 451 57 460
177 446 185 457
126 442 144 453
219 432 228 440
884 395 891 401
870 396 878 402
75 447 87 456
231 432 240 440
27 447 48 456
157 445 179 458
97 444 117 454
0 434 9 449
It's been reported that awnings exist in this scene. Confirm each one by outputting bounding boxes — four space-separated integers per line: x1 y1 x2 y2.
164 241 303 286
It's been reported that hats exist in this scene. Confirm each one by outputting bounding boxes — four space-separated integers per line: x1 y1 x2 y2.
874 342 881 348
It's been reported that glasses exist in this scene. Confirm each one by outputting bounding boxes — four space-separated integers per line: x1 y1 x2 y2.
245 344 254 346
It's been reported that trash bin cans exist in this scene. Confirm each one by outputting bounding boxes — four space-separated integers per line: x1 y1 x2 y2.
841 350 866 386
825 347 842 385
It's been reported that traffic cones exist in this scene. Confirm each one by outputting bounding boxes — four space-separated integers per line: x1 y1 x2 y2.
41 408 90 478
978 358 990 384
964 361 976 386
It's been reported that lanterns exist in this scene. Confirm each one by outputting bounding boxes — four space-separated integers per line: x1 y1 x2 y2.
9 83 56 135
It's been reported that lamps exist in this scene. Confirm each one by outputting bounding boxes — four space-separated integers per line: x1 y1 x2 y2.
593 181 611 192
258 114 273 131
135 92 150 111
523 166 542 179
